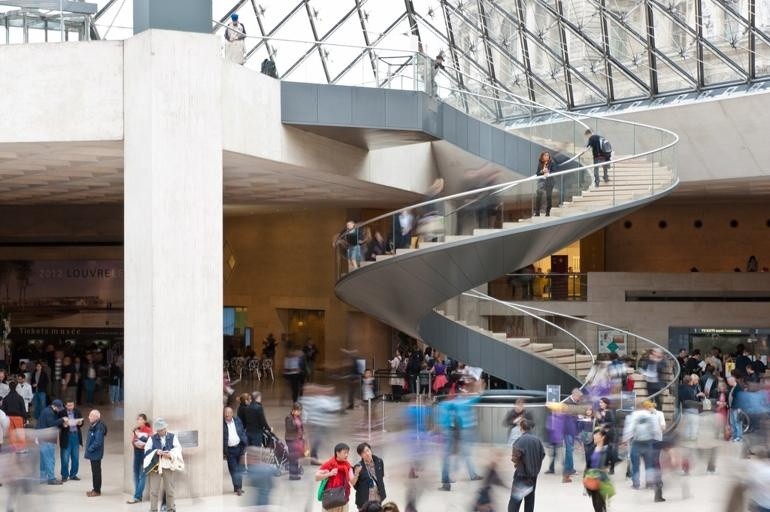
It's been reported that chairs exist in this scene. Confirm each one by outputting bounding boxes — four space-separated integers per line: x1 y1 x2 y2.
224 356 275 381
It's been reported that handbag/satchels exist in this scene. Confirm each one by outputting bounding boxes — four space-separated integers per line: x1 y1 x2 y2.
318 477 346 508
396 361 406 376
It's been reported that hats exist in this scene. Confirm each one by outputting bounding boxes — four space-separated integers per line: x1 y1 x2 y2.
153 417 168 431
52 399 65 411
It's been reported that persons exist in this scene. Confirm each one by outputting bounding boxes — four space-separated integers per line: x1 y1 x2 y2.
534 151 556 217
746 255 757 272
225 13 247 65
584 128 612 187
421 55 446 98
0 335 770 512
568 266 579 278
510 263 551 301
331 159 504 268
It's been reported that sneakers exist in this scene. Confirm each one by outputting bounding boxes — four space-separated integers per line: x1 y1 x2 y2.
236 487 245 496
86 490 101 497
607 467 667 502
409 471 484 490
544 468 577 482
289 475 302 480
39 476 81 485
